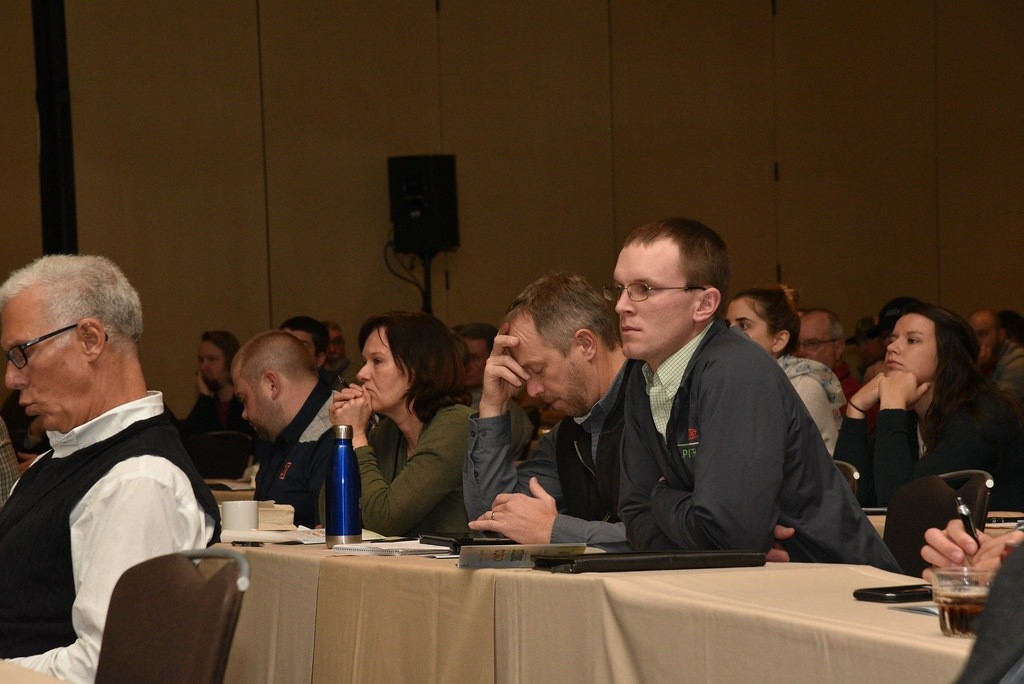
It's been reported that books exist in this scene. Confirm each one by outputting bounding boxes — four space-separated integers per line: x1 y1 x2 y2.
456 545 586 569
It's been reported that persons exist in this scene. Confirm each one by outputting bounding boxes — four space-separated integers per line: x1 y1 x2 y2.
461 274 627 545
723 285 1024 524
920 519 1024 684
450 323 534 461
0 255 222 684
602 218 903 574
177 316 379 530
328 311 479 539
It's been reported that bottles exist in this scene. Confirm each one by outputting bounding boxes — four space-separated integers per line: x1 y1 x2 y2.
325 423 363 549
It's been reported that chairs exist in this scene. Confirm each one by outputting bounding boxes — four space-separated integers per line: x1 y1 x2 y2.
885 469 995 577
90 549 248 684
190 429 252 480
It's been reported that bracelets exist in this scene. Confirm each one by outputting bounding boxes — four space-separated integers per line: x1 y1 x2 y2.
848 401 867 413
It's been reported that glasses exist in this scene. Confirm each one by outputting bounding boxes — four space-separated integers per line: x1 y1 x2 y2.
4 323 108 369
804 338 837 351
603 282 708 302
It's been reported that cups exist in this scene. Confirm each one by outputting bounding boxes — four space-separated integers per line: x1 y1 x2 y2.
932 566 993 639
222 499 258 531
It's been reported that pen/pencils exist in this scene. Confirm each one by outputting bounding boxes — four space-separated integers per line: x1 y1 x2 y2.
232 540 264 547
957 496 982 551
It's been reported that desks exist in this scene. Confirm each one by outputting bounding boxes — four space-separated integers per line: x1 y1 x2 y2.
205 543 977 684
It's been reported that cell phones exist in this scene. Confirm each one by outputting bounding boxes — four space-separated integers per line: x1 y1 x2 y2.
853 584 932 603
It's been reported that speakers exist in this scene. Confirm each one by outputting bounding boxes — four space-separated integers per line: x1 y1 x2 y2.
387 153 459 253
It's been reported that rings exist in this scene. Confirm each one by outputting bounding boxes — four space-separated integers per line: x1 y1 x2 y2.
491 511 498 519
